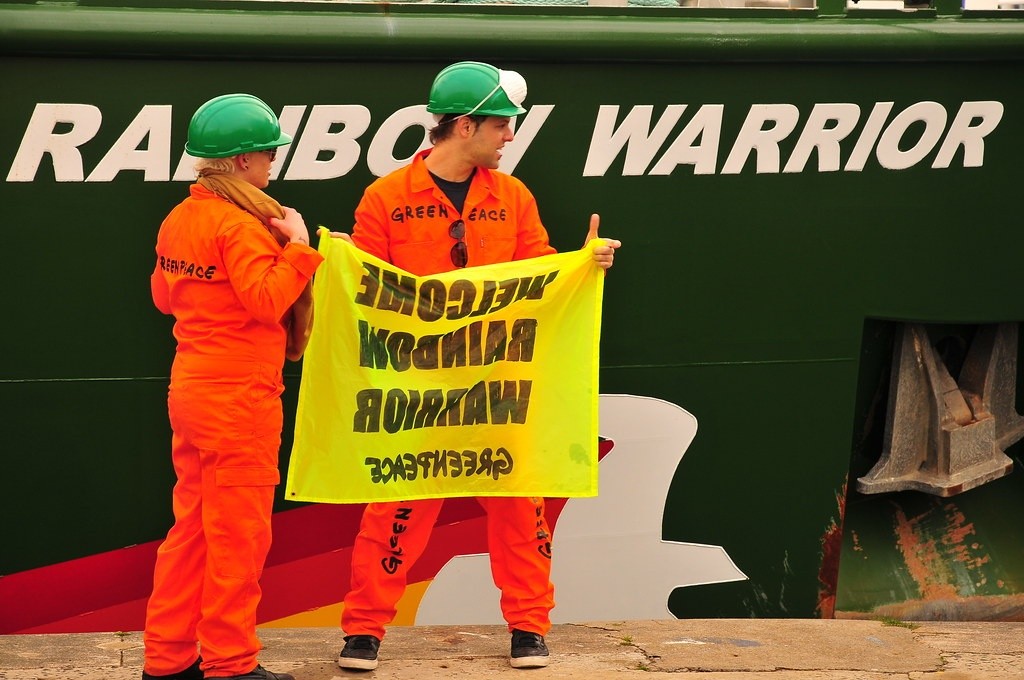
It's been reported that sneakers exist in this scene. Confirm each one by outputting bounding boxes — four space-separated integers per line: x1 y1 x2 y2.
338 635 380 669
510 628 549 667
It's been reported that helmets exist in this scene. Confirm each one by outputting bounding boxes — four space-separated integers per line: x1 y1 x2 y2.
426 61 528 117
185 93 293 158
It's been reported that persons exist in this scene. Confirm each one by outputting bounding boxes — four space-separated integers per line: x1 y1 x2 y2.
316 60 621 670
141 92 324 680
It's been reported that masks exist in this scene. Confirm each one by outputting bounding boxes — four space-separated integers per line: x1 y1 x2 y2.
441 68 528 124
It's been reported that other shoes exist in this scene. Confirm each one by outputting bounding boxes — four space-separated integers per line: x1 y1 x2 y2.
204 663 296 680
142 655 205 680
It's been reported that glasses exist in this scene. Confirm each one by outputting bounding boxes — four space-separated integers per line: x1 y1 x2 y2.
259 147 277 162
448 219 469 268
